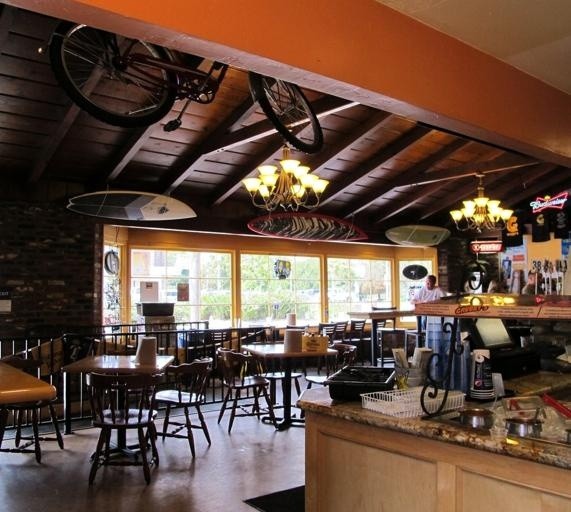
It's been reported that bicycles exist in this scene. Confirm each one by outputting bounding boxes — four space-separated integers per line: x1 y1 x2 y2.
38 20 323 154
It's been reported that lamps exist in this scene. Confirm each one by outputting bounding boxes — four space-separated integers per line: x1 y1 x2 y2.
448 168 513 233
243 75 330 212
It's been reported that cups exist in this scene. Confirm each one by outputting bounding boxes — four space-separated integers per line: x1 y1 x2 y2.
469 349 506 401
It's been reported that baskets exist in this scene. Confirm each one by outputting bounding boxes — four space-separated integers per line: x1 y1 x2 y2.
360 387 468 417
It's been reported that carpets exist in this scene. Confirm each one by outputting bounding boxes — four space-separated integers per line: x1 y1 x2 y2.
243 484 305 511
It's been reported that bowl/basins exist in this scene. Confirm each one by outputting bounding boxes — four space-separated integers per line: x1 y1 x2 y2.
458 409 545 440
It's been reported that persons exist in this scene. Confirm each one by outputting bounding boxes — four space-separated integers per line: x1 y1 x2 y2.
487 279 507 293
501 260 512 281
411 274 447 331
521 270 545 297
